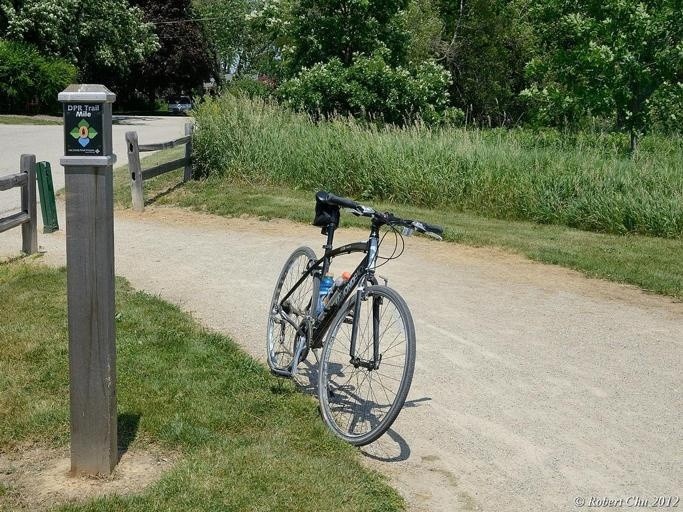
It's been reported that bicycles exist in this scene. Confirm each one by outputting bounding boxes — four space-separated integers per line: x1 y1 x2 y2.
266 191 445 447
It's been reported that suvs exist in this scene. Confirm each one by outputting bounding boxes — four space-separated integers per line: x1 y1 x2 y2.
167 95 191 113
